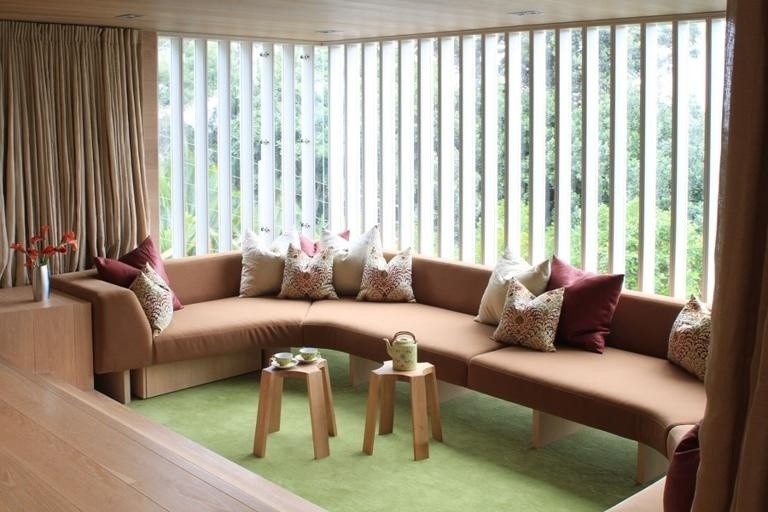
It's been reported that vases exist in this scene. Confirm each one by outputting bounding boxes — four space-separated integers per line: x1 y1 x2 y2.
32 265 50 301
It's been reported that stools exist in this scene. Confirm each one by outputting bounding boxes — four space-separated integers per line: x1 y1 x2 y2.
364 359 443 462
254 357 337 461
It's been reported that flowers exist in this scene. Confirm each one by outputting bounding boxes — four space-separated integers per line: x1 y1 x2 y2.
9 225 78 267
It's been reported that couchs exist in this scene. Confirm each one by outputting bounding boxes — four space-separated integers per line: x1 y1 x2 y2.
47 227 713 510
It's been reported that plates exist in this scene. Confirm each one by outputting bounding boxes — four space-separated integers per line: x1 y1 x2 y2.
295 355 318 363
271 359 299 369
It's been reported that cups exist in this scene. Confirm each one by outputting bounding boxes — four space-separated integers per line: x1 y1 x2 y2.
300 348 321 359
275 352 294 366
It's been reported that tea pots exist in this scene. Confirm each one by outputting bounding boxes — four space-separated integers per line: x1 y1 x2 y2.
383 331 417 371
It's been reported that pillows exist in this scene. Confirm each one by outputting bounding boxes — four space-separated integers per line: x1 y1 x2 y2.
94 235 184 340
239 227 414 301
666 295 710 383
472 246 625 353
